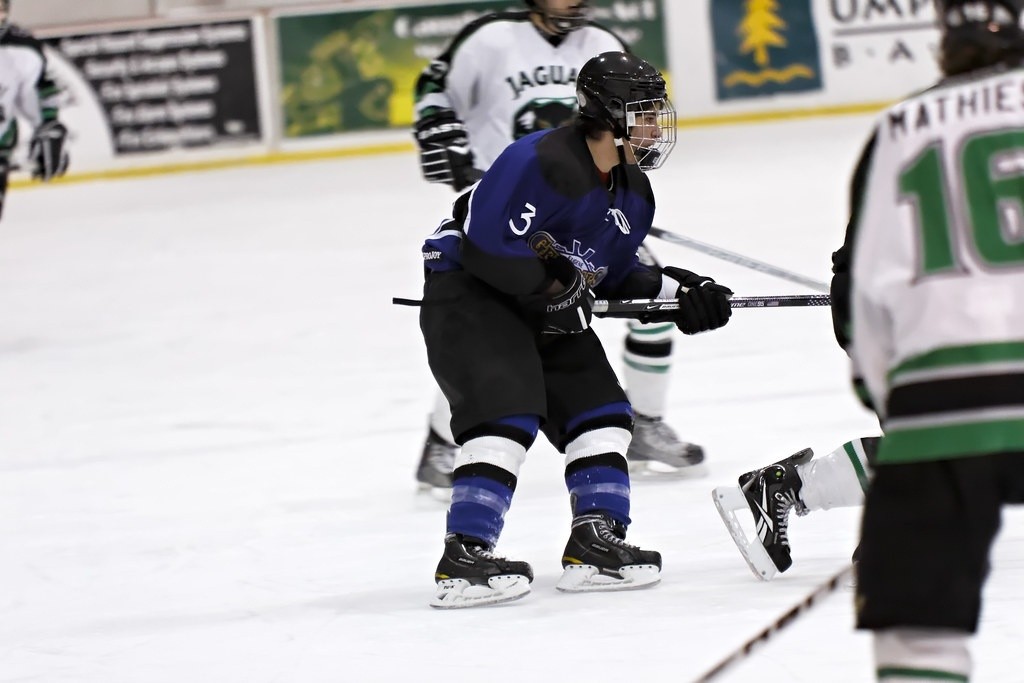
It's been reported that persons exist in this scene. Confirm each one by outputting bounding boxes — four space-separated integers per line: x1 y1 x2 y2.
713 123 884 584
411 0 704 488
850 0 1024 683
419 52 734 610
0 0 117 225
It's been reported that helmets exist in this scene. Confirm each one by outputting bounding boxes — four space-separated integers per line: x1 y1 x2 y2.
575 51 677 171
932 0 1024 58
525 0 592 35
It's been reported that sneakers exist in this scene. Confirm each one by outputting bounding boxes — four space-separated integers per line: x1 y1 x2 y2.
556 491 662 592
416 428 455 502
626 408 709 479
711 447 814 580
429 510 534 609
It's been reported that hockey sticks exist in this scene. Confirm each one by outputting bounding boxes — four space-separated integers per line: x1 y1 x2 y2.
381 291 832 316
643 226 831 290
694 560 854 683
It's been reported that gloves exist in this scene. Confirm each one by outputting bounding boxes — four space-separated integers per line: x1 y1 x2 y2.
541 267 595 335
27 122 70 181
674 275 733 334
413 110 474 192
